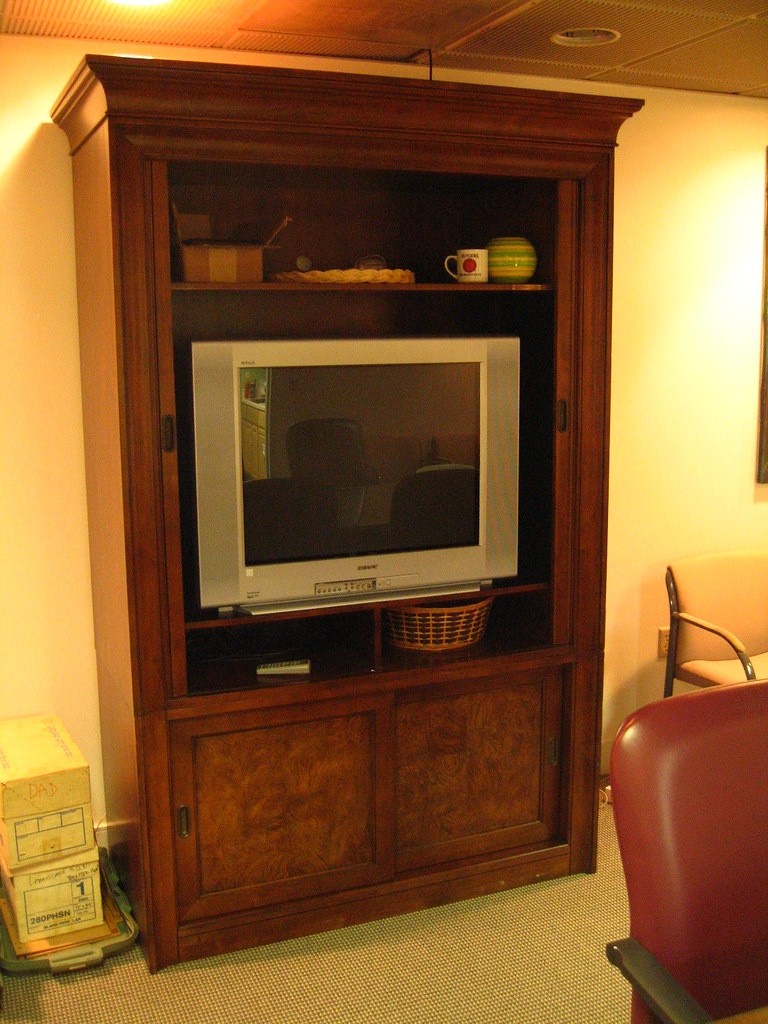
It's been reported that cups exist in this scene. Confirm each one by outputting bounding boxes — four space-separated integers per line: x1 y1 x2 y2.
445 249 489 283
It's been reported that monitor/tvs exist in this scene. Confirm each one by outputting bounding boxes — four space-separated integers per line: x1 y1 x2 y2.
189 342 519 616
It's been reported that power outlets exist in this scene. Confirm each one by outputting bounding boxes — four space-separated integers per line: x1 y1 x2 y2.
658 626 669 658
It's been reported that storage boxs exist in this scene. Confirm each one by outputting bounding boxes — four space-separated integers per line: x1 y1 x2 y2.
168 202 293 282
0 712 104 943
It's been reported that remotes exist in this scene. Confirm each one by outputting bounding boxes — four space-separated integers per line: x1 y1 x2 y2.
256 660 310 676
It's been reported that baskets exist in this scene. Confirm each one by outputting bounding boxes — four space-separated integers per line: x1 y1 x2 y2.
382 596 498 652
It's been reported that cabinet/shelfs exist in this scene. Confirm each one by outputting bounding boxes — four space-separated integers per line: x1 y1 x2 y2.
51 52 647 987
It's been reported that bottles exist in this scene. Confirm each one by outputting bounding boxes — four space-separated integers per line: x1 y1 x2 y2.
485 237 537 284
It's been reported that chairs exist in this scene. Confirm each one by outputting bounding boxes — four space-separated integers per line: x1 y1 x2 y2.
664 550 768 699
604 678 768 1024
